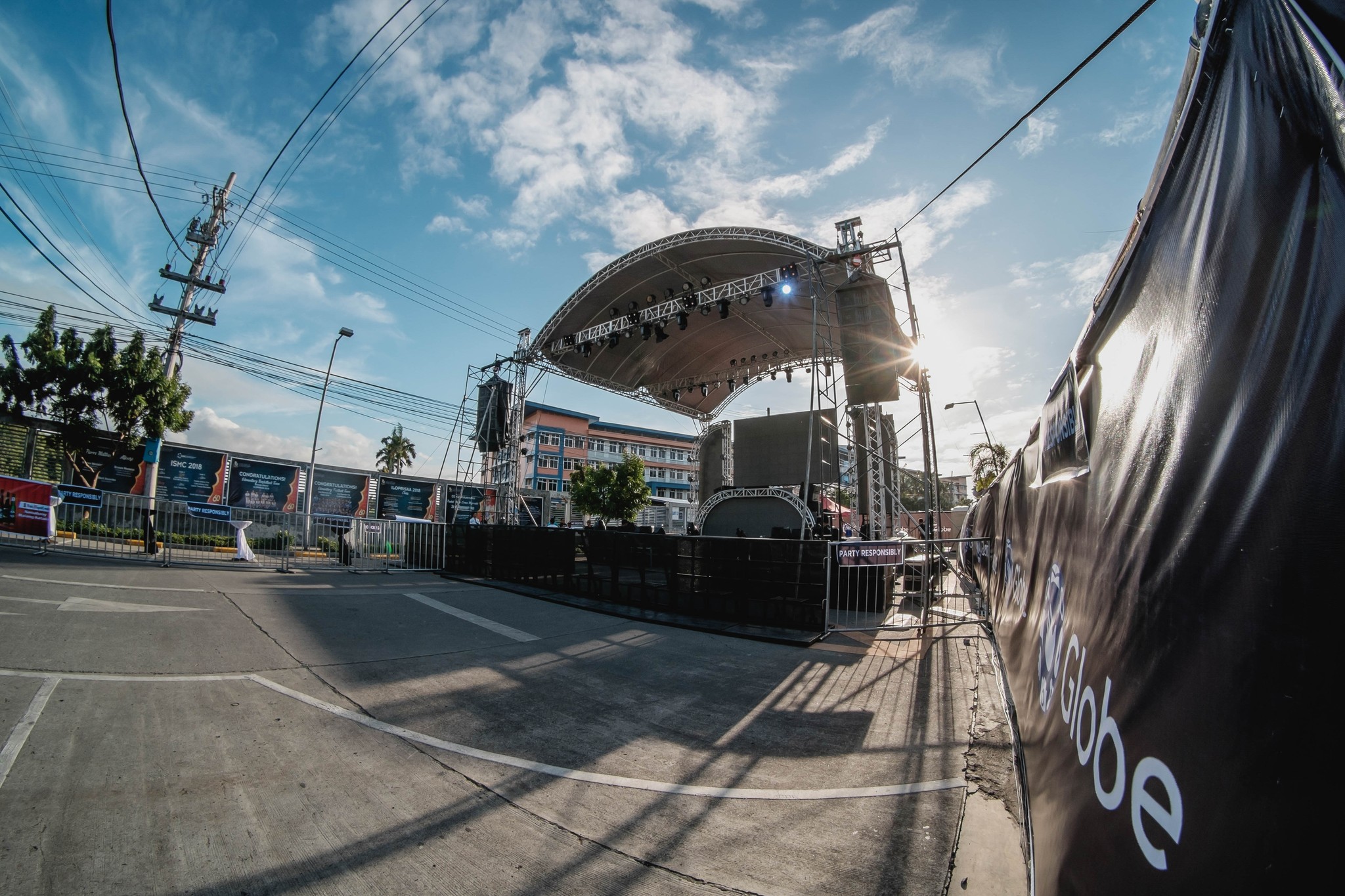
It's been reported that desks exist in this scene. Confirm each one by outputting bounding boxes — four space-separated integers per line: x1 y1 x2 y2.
229 520 255 562
36 495 63 544
135 507 159 555
331 527 352 566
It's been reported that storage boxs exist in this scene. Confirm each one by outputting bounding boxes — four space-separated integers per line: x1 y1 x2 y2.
902 554 940 593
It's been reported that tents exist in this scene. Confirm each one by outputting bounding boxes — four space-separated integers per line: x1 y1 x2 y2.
819 494 856 518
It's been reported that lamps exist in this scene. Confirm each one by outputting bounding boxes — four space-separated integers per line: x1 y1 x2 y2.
736 527 746 536
687 457 693 462
847 467 854 474
693 436 699 441
881 416 886 422
846 445 853 451
520 447 528 456
720 454 727 461
721 475 727 481
562 261 810 402
771 526 813 540
687 522 700 536
848 491 855 499
891 466 895 470
688 497 694 503
721 433 728 439
688 478 694 482
848 407 862 420
889 438 895 446
519 435 527 443
892 490 898 498
846 422 851 428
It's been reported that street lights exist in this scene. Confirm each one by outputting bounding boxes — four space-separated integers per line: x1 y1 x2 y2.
943 399 998 475
303 327 354 552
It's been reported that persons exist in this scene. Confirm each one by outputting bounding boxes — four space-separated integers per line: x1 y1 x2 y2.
595 520 605 530
481 517 487 524
617 519 629 532
547 518 558 527
312 494 352 516
560 518 568 527
469 512 480 524
918 518 925 540
823 517 833 541
245 489 278 510
481 496 495 524
584 520 594 530
547 516 559 527
